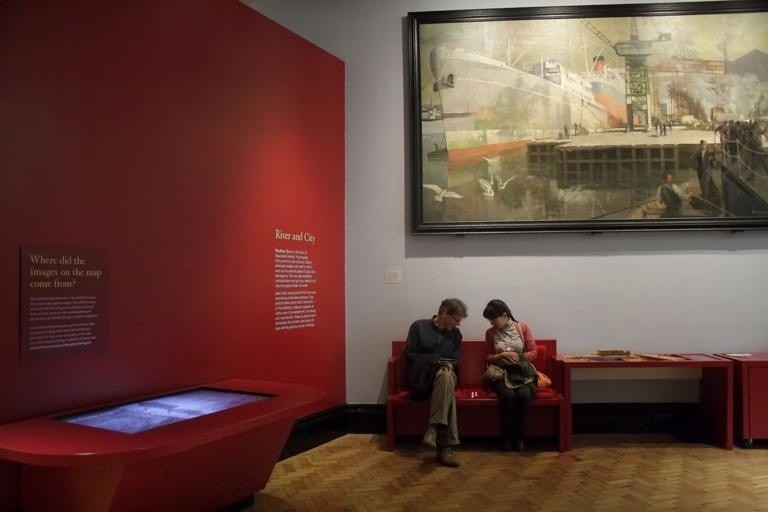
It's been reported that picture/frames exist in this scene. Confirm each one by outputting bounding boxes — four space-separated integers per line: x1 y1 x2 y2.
403 1 768 235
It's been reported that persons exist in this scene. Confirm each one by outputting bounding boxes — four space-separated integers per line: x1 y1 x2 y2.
402 297 469 469
559 122 589 140
483 298 538 454
654 117 768 215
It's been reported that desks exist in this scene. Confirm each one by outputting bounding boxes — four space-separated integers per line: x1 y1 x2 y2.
559 351 735 451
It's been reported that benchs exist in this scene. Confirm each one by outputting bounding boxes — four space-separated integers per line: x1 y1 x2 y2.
387 340 574 452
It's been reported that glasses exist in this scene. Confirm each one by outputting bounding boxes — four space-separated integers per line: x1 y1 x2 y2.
449 313 461 322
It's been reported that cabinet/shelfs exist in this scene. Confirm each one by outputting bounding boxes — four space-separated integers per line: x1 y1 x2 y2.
714 352 768 447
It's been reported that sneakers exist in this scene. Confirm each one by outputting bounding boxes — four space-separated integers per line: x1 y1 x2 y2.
437 445 460 467
423 426 438 448
503 438 524 452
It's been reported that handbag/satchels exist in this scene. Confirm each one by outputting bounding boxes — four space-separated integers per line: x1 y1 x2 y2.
483 364 503 383
